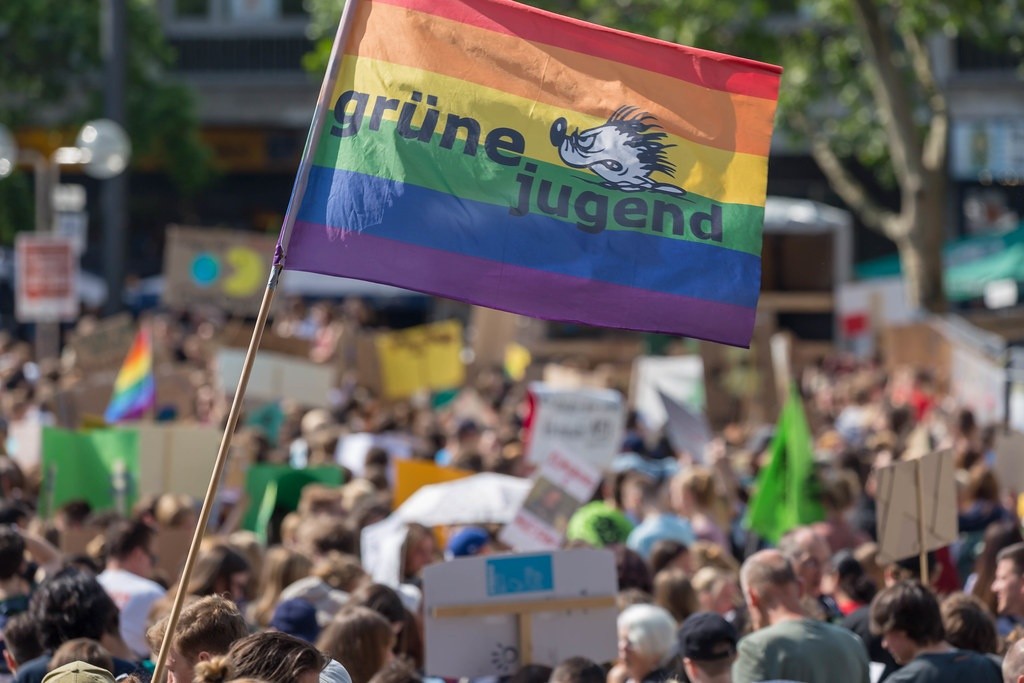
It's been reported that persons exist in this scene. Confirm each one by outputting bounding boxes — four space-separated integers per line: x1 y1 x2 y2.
1 299 1022 683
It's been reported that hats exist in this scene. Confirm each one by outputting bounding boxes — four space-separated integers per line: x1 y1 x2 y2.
274 598 315 635
281 576 348 623
42 660 114 683
679 613 737 660
452 529 488 556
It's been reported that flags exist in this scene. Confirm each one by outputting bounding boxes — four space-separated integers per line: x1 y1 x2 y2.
282 0 783 356
